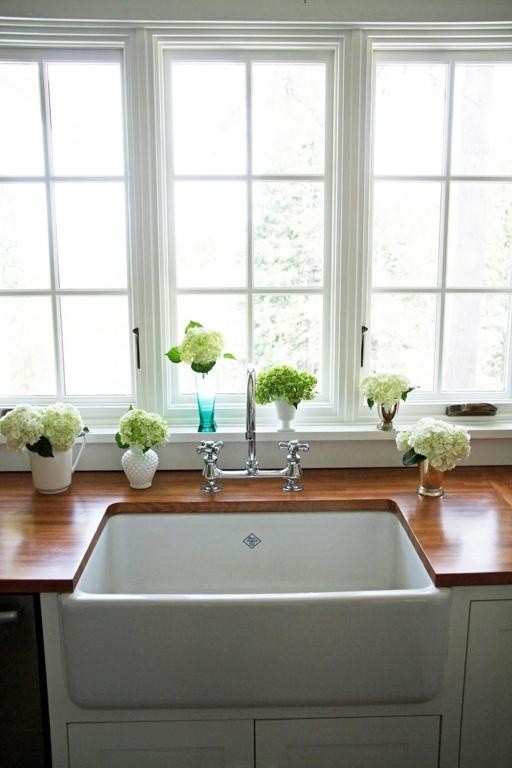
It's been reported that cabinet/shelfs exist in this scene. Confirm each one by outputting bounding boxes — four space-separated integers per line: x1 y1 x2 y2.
0 584 512 767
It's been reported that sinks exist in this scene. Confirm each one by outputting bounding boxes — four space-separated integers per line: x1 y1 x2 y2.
56 506 455 709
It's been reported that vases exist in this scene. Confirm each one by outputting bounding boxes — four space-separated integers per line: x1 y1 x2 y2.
120 448 159 489
375 400 398 432
416 459 444 497
273 399 296 432
195 374 218 432
29 450 73 497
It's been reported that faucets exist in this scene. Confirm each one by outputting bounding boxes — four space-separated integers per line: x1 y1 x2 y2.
242 369 259 476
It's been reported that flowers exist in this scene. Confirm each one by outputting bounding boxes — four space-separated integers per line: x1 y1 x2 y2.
253 365 318 410
116 404 167 454
0 403 85 459
163 320 237 373
360 373 421 412
394 416 472 472
